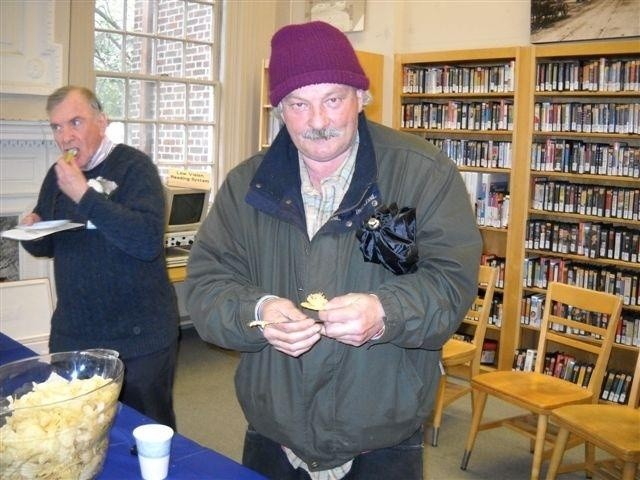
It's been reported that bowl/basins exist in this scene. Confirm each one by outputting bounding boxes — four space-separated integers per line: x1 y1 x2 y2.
0 347 126 479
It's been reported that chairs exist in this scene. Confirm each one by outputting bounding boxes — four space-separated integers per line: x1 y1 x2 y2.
461 282 623 479
545 355 640 479
426 265 498 446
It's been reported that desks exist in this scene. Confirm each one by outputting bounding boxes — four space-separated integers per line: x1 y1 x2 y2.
0 332 277 480
166 247 200 331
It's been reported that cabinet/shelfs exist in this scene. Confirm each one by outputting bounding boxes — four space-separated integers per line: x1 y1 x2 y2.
393 39 640 405
259 48 384 153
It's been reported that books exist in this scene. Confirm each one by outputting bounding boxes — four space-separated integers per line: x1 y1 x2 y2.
521 294 640 347
533 57 640 134
512 349 633 404
452 171 510 365
523 219 640 306
530 138 640 219
403 60 515 167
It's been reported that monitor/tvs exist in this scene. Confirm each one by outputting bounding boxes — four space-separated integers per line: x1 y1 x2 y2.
162 183 210 233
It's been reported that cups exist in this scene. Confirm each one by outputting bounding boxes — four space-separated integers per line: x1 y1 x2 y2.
132 425 173 480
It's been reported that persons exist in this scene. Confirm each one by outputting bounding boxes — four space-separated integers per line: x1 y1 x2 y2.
183 20 482 480
19 84 183 437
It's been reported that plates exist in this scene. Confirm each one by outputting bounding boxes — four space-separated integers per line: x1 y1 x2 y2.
17 220 70 231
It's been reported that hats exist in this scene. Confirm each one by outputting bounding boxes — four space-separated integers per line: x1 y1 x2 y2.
269 20 369 107
